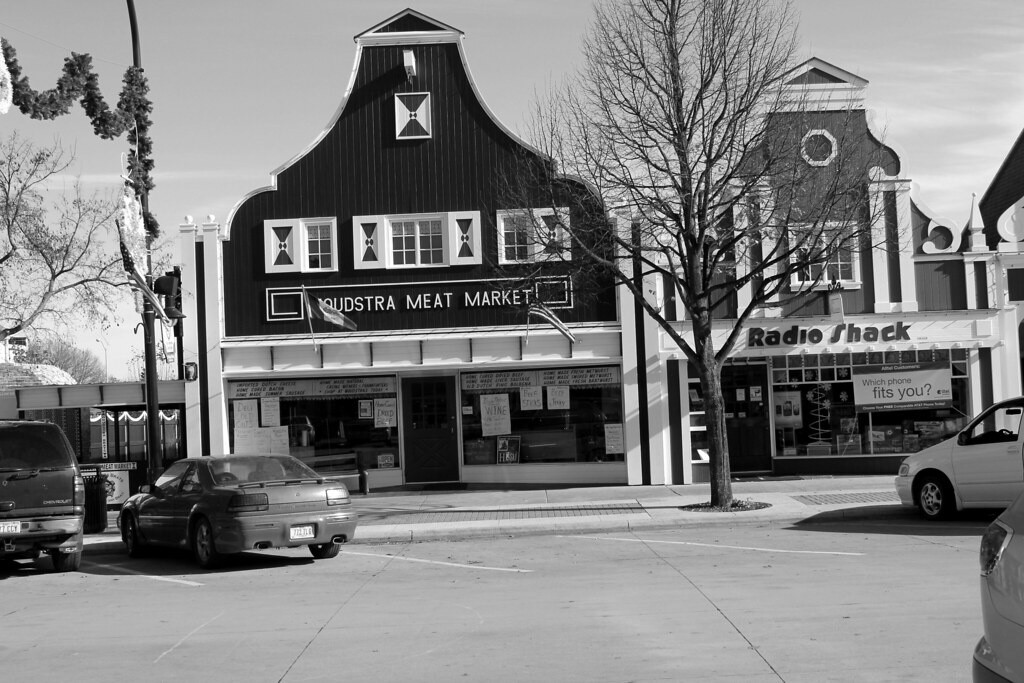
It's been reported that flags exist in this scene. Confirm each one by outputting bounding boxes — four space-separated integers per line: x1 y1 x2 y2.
528 296 574 343
304 290 357 330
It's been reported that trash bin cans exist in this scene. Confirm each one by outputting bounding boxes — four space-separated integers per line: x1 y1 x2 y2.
75 464 109 537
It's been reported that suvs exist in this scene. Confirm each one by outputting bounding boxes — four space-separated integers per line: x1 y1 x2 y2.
1 421 85 571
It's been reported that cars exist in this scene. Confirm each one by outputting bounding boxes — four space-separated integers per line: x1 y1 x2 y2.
895 394 1024 519
119 453 358 568
970 486 1024 683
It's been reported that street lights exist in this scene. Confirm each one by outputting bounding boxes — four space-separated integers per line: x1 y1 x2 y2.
132 274 189 497
